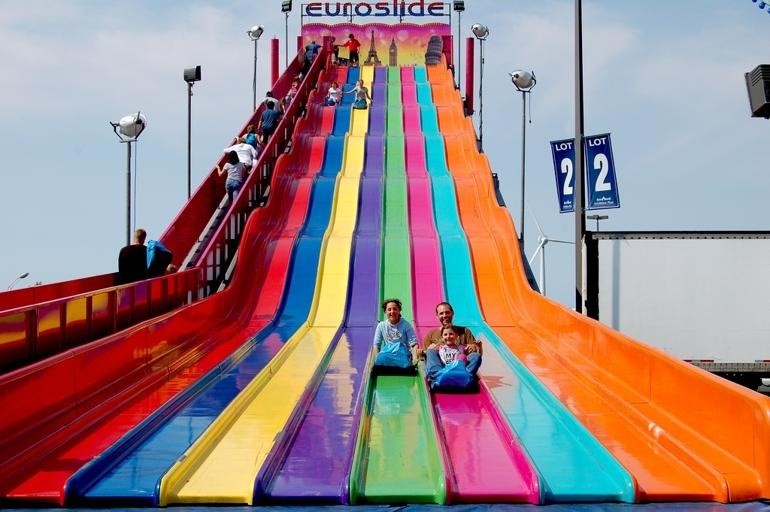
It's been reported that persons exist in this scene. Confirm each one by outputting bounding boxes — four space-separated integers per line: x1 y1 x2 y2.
372 298 418 373
344 80 373 109
324 81 343 106
145 239 172 277
215 41 323 204
332 33 361 67
117 229 147 285
415 302 483 392
428 325 473 366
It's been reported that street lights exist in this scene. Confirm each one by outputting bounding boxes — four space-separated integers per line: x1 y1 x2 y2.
470 23 490 146
184 65 201 200
109 110 148 247
245 26 264 112
745 64 770 120
508 70 537 251
281 1 292 70
8 272 29 292
587 215 608 232
454 0 465 90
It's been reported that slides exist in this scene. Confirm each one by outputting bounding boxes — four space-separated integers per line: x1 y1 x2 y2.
0 55 770 504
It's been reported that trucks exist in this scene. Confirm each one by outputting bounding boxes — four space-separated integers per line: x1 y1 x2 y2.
581 231 770 392
